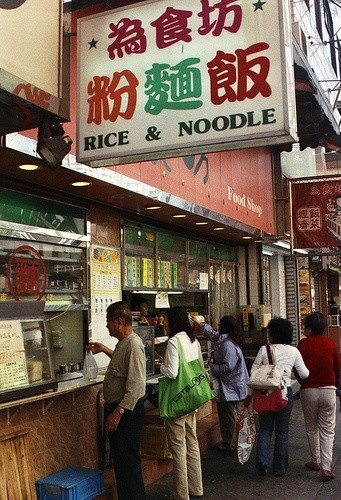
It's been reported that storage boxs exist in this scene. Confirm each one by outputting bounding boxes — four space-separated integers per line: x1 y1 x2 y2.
36 466 105 500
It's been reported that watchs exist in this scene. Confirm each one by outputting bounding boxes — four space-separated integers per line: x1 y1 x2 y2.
117 406 126 414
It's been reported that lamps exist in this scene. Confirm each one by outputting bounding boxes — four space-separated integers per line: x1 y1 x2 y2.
36 121 72 170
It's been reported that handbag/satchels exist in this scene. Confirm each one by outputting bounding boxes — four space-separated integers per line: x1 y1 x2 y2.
248 345 285 388
252 387 289 414
237 406 256 465
157 336 215 418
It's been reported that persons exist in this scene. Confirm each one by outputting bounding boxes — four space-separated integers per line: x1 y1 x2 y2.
86 302 147 500
133 297 167 327
189 314 249 450
293 312 341 479
160 308 204 500
251 318 309 476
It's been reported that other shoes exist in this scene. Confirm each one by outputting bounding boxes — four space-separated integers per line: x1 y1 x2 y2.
324 473 335 480
305 462 319 471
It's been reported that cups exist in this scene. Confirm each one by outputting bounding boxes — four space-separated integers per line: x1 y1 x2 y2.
86 366 98 379
59 362 83 374
